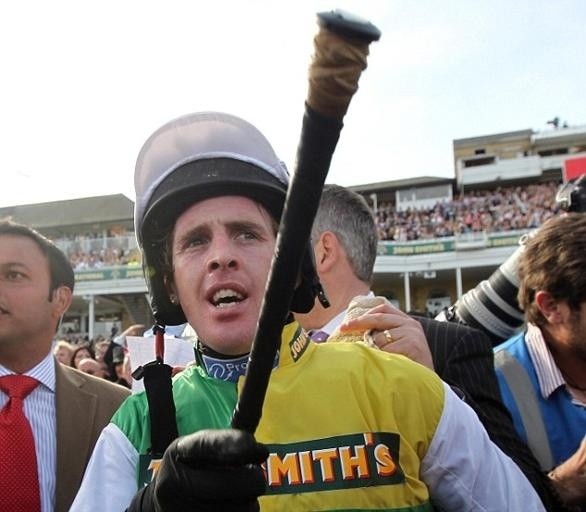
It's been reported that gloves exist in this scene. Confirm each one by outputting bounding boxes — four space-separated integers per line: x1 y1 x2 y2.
129 428 270 512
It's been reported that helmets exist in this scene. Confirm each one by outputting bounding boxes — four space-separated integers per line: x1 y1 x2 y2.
134 111 330 327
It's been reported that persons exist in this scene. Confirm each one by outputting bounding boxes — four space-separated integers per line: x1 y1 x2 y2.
56 323 147 389
375 180 564 242
293 185 564 511
492 213 586 505
67 228 143 271
70 110 546 511
0 221 132 512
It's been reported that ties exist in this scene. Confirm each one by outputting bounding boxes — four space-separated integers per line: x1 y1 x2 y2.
0 374 40 511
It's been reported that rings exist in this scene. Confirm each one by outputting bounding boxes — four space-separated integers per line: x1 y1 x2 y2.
383 329 393 342
